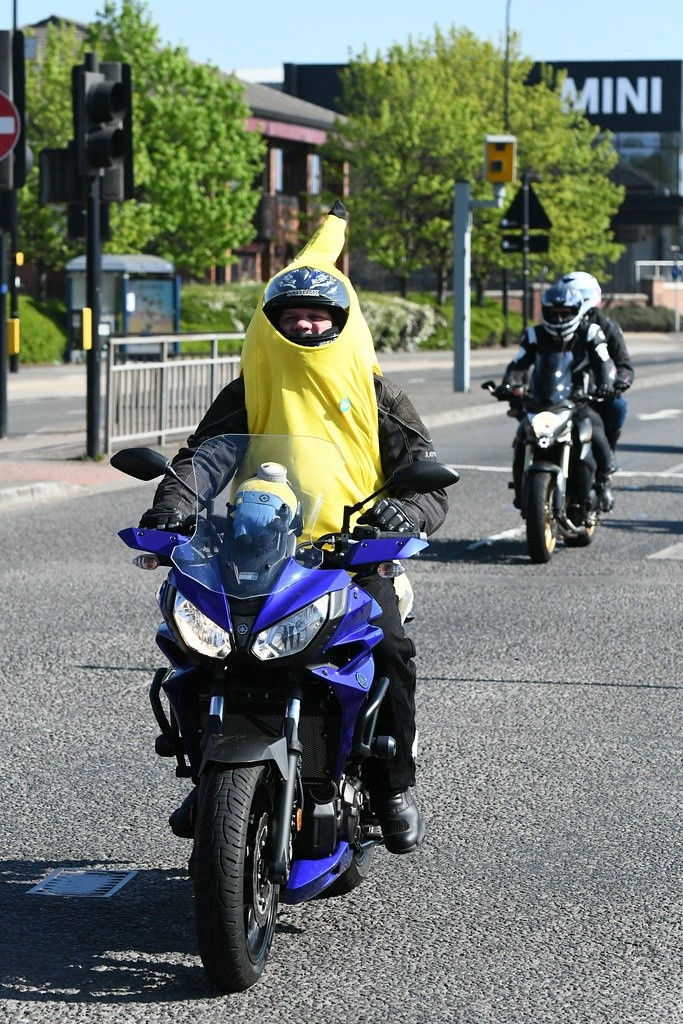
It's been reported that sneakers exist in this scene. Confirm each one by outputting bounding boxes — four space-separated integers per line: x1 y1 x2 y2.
379 787 424 853
595 480 617 512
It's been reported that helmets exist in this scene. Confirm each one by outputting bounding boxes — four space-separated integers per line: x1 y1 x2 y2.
262 268 350 348
540 273 600 336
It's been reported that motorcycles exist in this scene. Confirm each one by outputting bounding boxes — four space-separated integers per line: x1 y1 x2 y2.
107 426 460 992
481 349 620 563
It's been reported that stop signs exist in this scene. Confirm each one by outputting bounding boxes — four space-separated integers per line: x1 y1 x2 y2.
0 90 21 163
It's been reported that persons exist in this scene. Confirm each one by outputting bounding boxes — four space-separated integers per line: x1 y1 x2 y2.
559 272 632 442
501 284 616 514
140 265 447 854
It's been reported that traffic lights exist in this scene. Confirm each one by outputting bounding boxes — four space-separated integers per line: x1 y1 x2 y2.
76 69 132 179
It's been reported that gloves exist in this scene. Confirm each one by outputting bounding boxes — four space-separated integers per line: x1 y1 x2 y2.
596 384 613 397
494 383 516 398
362 499 414 532
139 508 187 534
